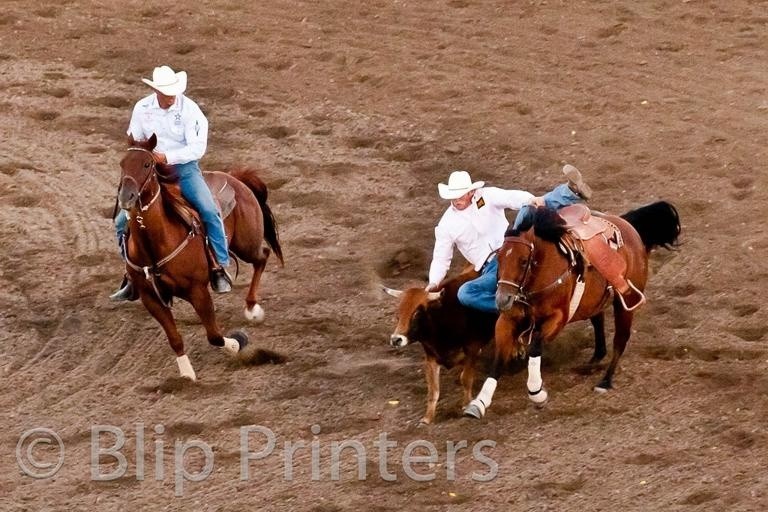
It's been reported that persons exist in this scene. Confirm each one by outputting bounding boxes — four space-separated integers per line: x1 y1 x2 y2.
108 64 233 301
425 164 592 313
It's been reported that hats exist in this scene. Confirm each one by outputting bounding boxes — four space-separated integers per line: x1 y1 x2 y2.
437 170 484 201
139 63 189 99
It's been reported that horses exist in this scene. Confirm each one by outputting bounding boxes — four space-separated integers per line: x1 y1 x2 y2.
117 132 285 382
462 201 685 420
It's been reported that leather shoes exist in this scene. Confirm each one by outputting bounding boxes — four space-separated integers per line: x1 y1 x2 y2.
218 272 233 294
107 280 140 302
561 163 593 200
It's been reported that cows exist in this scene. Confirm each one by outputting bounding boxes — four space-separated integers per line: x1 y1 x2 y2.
378 267 527 429
